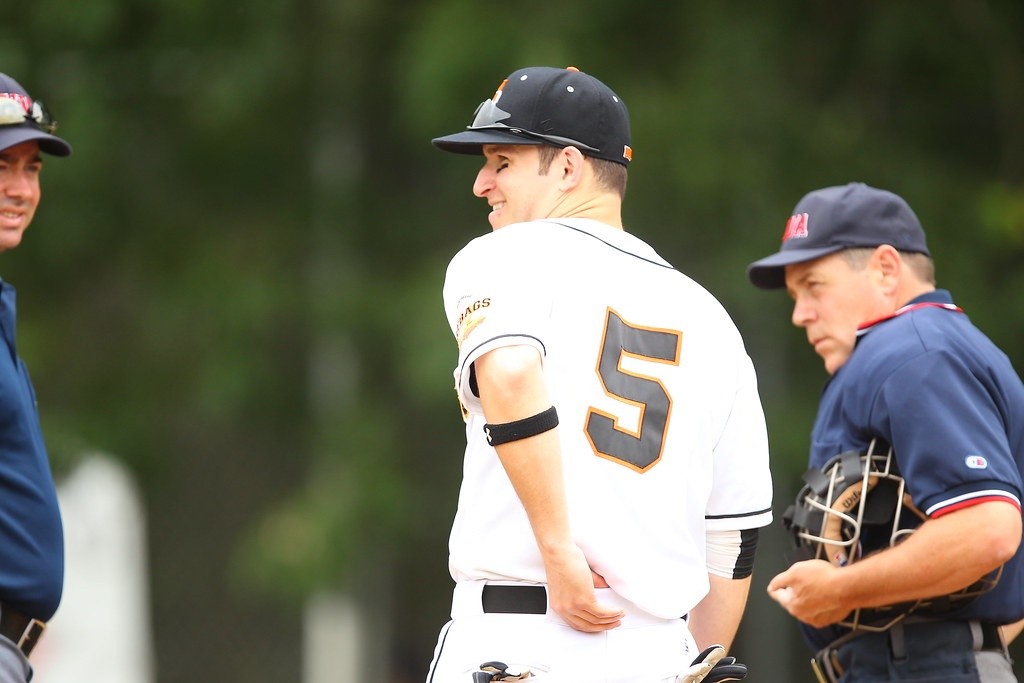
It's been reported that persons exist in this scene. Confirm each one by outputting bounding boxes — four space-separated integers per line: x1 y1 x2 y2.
746 181 1024 683
421 67 774 683
0 72 72 683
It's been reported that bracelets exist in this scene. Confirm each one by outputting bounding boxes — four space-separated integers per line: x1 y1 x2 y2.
484 406 559 447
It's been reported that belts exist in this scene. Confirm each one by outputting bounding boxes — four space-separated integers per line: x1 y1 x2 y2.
0 608 45 657
480 582 691 620
810 621 1004 683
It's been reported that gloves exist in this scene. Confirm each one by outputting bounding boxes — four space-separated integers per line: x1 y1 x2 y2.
676 645 747 683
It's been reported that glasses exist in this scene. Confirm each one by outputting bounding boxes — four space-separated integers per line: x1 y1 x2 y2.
0 98 53 133
467 99 600 153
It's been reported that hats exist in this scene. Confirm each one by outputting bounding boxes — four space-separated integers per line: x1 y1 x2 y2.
746 182 930 290
431 66 632 167
0 72 72 157
